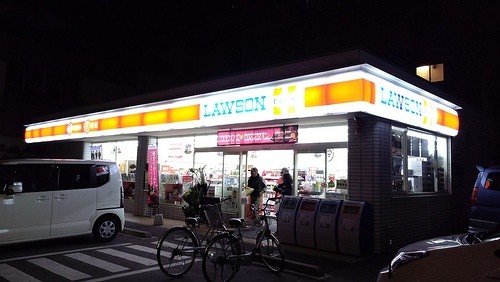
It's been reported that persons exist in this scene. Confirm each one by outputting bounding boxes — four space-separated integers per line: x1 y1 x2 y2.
248 168 267 228
272 167 292 238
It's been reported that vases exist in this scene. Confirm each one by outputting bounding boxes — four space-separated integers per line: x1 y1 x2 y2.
184 208 197 223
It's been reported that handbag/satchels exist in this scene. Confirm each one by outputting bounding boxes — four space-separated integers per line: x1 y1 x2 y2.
244 188 255 195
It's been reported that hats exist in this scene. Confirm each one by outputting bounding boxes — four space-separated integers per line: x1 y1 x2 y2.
248 167 258 172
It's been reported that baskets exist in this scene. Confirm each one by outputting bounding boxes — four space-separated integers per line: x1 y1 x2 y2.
204 207 221 226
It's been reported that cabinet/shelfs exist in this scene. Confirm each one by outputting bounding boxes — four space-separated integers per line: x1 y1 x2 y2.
422 160 445 192
260 176 280 211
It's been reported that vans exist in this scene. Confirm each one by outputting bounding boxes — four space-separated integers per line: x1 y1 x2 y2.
0 157 125 245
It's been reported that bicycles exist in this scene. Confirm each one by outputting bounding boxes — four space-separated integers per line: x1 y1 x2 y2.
155 197 235 277
201 196 286 282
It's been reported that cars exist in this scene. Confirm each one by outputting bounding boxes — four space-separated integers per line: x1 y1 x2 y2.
377 232 500 282
468 165 500 233
